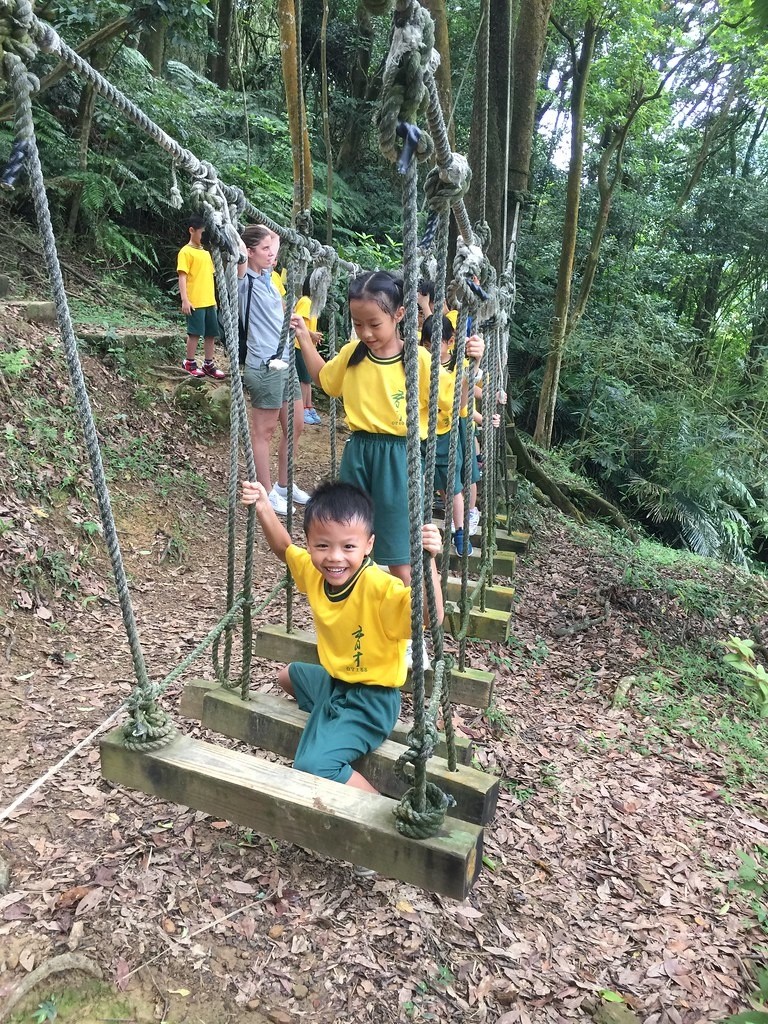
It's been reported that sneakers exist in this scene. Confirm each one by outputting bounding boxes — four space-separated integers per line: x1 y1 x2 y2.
468 506 480 536
303 408 322 425
273 481 312 504
442 507 456 534
181 358 206 378
201 361 226 379
451 526 474 559
266 489 297 516
406 638 430 671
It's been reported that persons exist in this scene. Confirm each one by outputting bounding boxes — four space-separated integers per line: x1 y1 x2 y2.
177 214 325 513
241 479 443 797
290 271 507 670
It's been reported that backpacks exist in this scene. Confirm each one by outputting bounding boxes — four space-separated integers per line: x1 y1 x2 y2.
213 273 254 366
